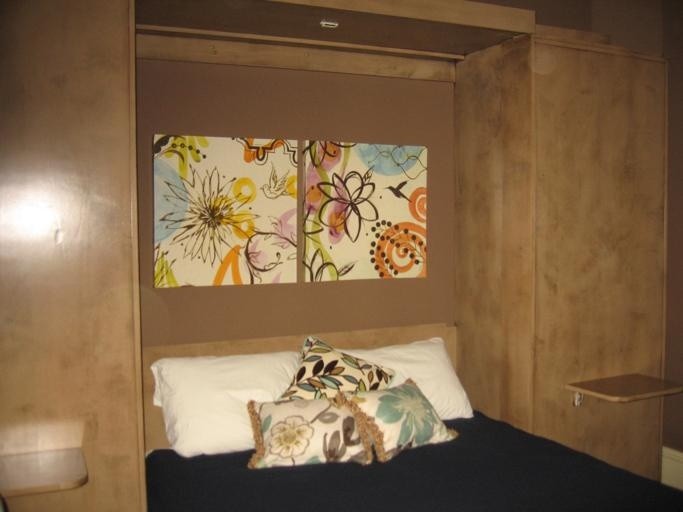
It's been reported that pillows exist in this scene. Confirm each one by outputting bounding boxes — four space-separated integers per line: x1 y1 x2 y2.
333 377 459 463
150 350 304 459
331 334 475 422
246 397 374 469
277 335 396 403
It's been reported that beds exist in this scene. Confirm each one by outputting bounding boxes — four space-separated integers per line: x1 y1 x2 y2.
141 320 683 510
